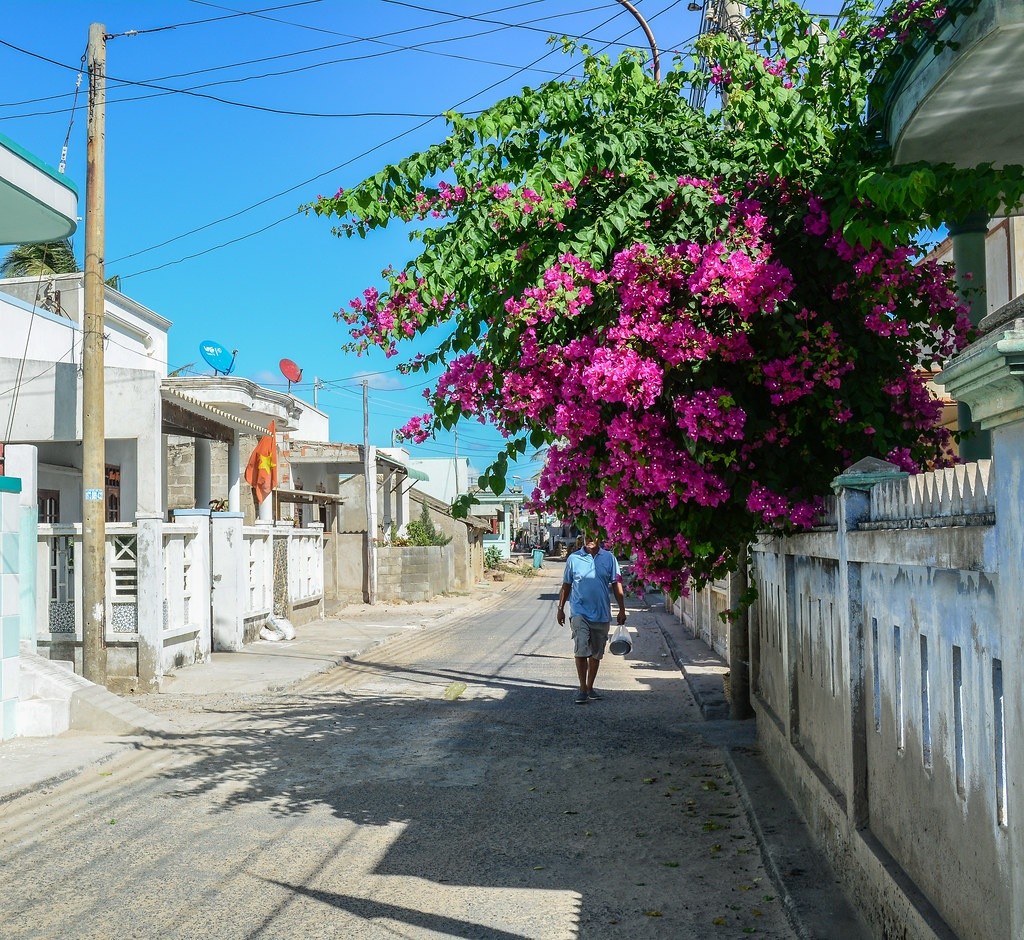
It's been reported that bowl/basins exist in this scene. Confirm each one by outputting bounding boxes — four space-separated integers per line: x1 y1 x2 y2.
610 640 631 656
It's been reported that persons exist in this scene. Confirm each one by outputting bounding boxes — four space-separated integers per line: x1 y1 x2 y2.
558 523 627 702
530 545 534 549
515 535 520 545
576 535 582 551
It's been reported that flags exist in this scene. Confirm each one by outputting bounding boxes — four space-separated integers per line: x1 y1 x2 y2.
244 418 277 505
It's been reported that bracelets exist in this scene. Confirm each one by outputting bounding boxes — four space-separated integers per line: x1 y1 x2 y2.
558 606 563 610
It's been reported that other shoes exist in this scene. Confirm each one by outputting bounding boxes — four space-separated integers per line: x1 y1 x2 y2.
588 691 602 700
574 688 588 703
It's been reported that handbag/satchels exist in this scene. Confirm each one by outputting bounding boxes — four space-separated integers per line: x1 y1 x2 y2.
610 622 633 653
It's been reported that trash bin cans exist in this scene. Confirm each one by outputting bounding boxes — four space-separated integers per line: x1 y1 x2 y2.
531 548 546 568
